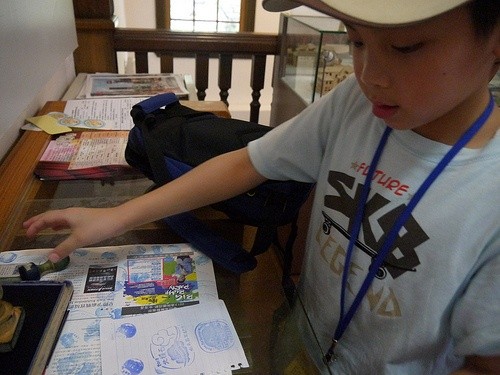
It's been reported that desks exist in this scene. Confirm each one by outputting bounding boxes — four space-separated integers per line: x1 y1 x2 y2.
0 101 332 375
60 72 189 101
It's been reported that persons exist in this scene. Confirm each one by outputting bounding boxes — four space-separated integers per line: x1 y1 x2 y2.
23 0 500 375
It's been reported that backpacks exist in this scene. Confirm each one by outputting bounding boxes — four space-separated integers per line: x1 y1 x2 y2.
123 91 311 269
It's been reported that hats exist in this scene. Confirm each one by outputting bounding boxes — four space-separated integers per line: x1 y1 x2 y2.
261 0 472 26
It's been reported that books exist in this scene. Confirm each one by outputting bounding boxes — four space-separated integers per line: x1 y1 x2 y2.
0 280 73 375
